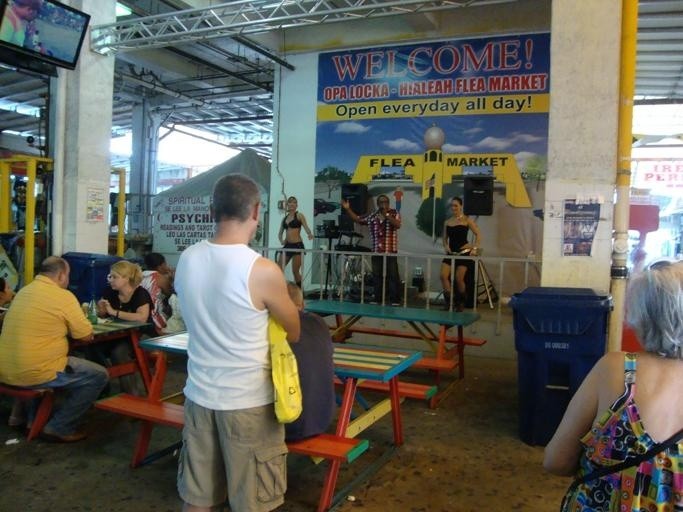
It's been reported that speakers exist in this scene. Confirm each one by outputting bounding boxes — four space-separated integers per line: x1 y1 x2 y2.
341 183 368 216
464 175 494 216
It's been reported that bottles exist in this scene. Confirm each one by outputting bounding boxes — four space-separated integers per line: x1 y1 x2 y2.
81 292 98 324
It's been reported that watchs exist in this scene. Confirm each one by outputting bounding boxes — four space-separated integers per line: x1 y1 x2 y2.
384 212 389 217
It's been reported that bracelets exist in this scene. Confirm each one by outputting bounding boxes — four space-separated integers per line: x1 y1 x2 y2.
472 246 478 250
306 230 311 235
115 309 120 320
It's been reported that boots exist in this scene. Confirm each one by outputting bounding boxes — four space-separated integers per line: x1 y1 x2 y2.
439 289 454 312
454 288 468 312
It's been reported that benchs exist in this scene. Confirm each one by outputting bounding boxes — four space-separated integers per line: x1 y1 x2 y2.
296 298 494 409
325 370 439 445
92 392 371 510
1 379 62 445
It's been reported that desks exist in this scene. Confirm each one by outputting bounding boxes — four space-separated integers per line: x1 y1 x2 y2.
129 330 424 511
0 303 157 445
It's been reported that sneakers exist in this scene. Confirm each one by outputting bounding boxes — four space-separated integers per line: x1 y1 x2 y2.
7 414 33 431
390 299 401 306
367 297 388 304
39 426 88 444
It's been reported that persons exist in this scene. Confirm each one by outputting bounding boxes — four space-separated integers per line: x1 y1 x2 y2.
542 265 683 510
0 277 17 331
439 197 480 312
284 280 334 443
0 256 109 441
0 0 43 53
174 172 300 512
276 196 313 298
138 252 175 336
392 185 403 215
339 194 400 306
86 260 154 396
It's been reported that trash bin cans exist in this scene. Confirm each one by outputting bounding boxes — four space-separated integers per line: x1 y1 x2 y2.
507 286 613 448
60 251 125 319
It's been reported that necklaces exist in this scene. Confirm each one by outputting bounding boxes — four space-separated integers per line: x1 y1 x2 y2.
118 288 133 309
452 214 464 220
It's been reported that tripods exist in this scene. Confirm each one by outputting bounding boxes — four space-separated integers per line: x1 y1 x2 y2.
476 216 499 308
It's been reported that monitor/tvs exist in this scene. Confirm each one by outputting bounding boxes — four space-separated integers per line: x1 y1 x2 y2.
0 0 91 70
